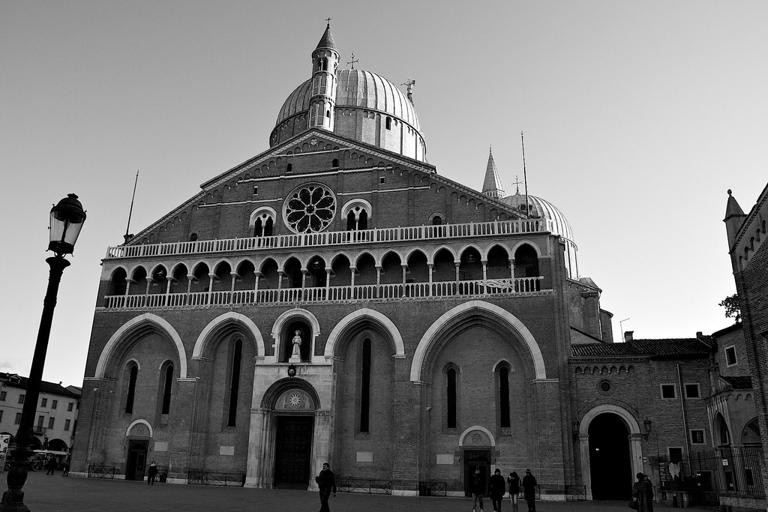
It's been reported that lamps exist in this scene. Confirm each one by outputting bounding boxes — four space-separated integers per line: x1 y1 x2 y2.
571 415 580 439
643 416 652 438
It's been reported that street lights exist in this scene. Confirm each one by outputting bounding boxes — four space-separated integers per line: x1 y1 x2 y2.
0 193 86 512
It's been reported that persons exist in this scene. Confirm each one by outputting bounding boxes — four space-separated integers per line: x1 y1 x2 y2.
470 469 536 512
148 462 157 486
316 463 336 512
633 473 653 512
292 330 301 354
46 455 56 475
